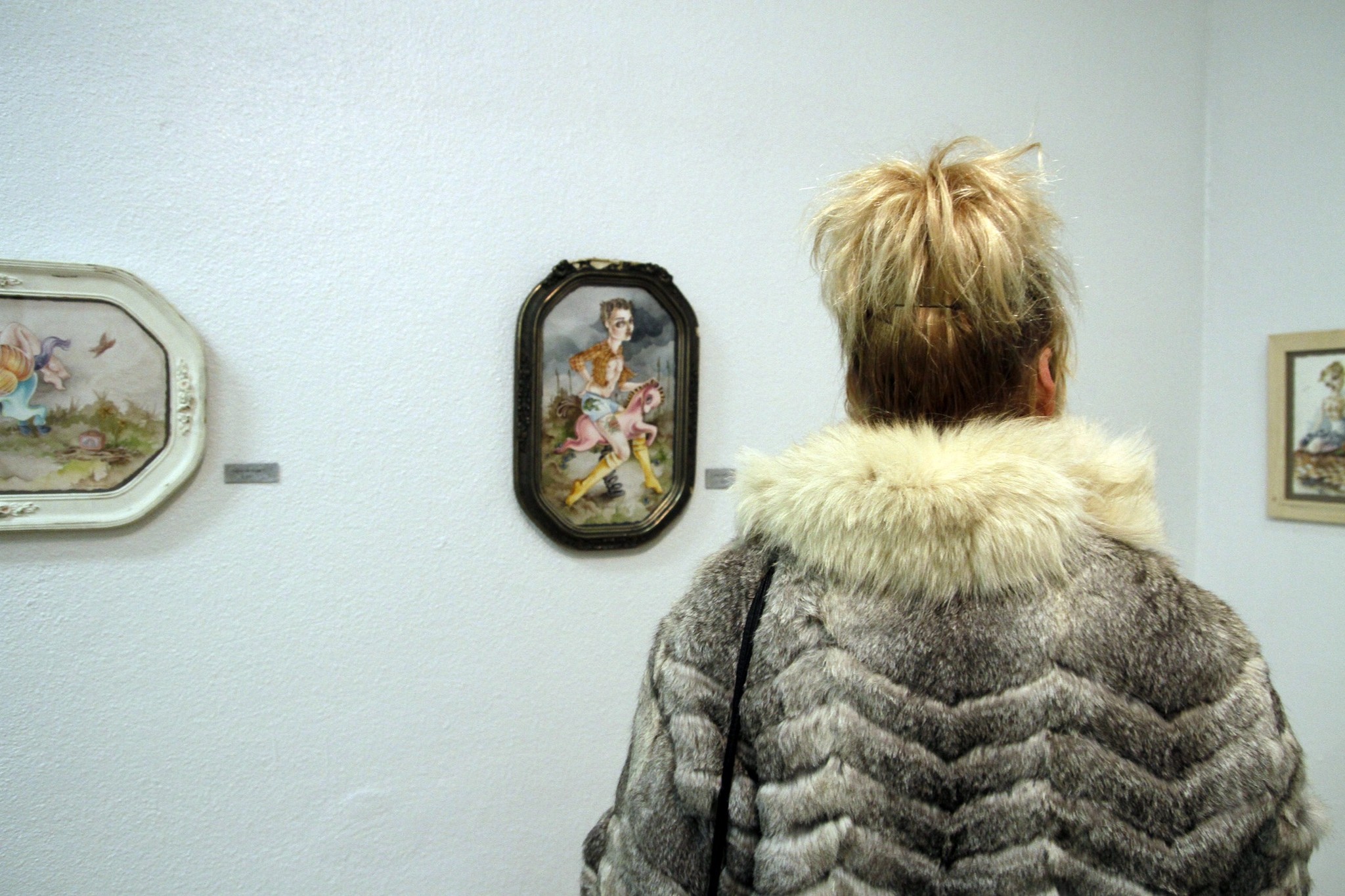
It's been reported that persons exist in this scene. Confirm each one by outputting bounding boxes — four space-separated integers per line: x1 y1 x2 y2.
580 134 1328 896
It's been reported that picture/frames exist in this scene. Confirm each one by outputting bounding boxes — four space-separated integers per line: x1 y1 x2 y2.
1 260 204 531
1265 329 1345 525
512 260 700 553
